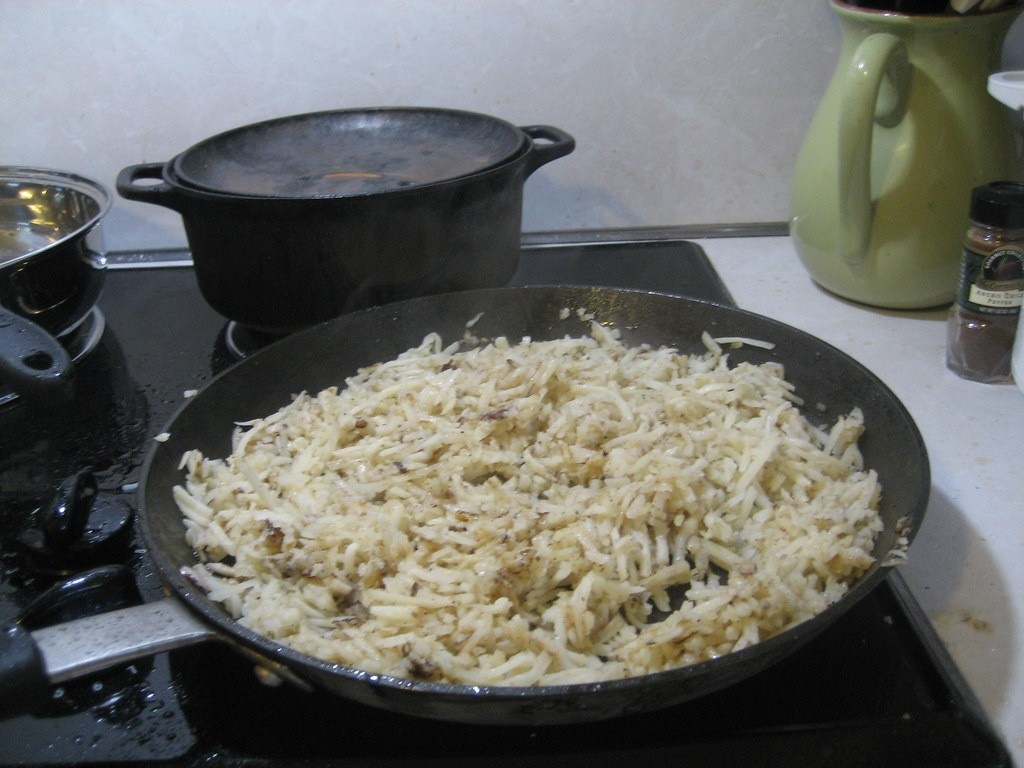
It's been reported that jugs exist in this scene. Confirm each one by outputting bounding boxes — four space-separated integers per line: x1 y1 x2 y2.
790 0 1024 308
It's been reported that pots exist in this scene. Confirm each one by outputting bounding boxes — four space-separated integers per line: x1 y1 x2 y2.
0 286 930 722
0 164 112 401
116 106 573 324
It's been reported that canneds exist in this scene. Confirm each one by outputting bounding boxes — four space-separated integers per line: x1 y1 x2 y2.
944 179 1024 382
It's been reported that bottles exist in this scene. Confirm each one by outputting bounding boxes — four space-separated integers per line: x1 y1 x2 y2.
945 182 1023 383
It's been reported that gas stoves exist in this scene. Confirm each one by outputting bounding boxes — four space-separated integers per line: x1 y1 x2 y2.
0 240 1014 768
